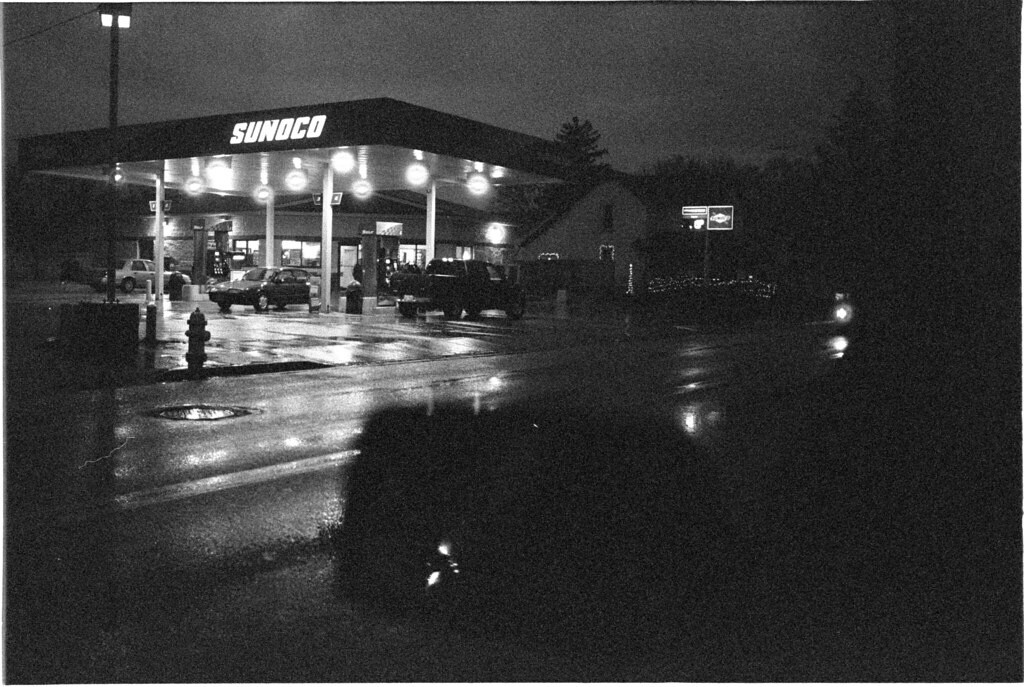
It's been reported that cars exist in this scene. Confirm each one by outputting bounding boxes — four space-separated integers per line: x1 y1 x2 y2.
209 265 316 312
93 258 191 294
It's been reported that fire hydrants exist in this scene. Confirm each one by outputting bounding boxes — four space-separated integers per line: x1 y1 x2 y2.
185 306 211 374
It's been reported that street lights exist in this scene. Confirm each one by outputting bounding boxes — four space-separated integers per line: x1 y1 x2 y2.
96 3 132 304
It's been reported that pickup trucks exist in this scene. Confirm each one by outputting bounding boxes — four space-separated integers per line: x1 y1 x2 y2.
390 260 527 321
